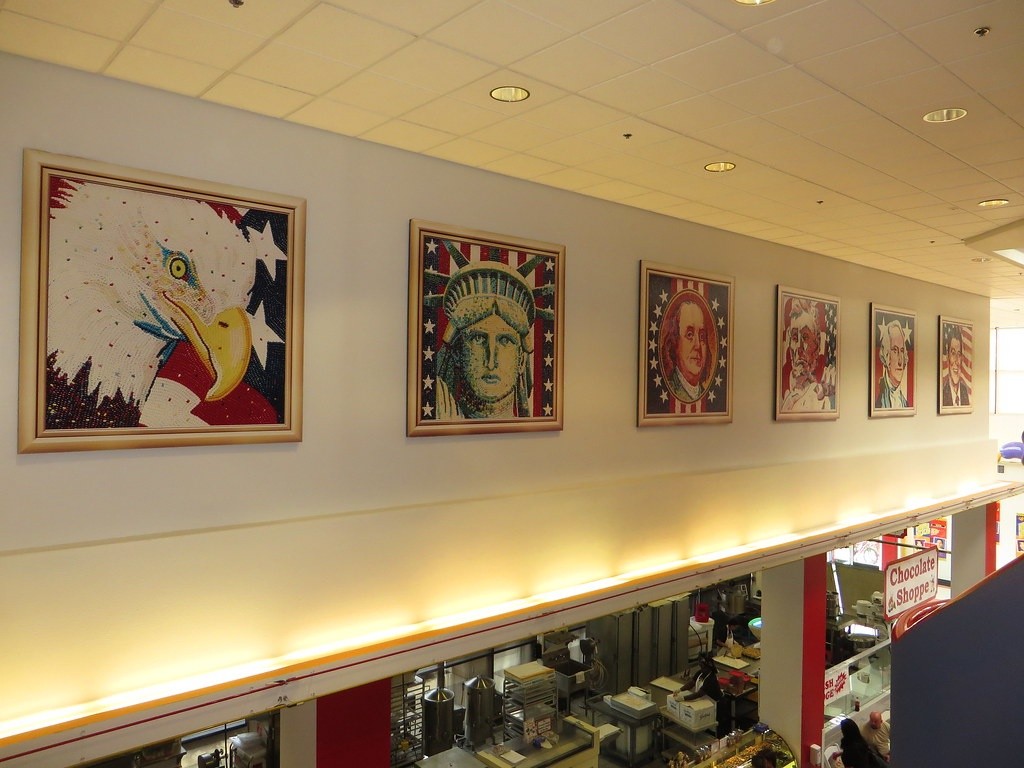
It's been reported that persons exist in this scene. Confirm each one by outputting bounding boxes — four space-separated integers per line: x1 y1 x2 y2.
838 709 890 768
673 653 732 740
712 618 739 657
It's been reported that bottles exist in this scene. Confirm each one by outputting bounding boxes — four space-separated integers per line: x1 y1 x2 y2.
851 695 854 710
854 697 860 712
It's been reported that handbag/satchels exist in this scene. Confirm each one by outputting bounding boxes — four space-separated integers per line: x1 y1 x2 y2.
695 603 710 623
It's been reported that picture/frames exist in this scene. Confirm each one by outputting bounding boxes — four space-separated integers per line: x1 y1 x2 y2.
407 219 565 436
870 302 917 419
18 149 306 454
939 315 975 415
776 285 842 421
638 259 734 428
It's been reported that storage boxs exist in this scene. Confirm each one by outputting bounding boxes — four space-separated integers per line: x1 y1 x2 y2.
679 695 715 727
666 690 701 719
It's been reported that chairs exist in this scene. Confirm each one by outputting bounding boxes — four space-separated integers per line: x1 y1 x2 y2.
824 710 890 768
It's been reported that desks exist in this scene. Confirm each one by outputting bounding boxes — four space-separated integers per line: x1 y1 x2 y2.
679 642 759 703
